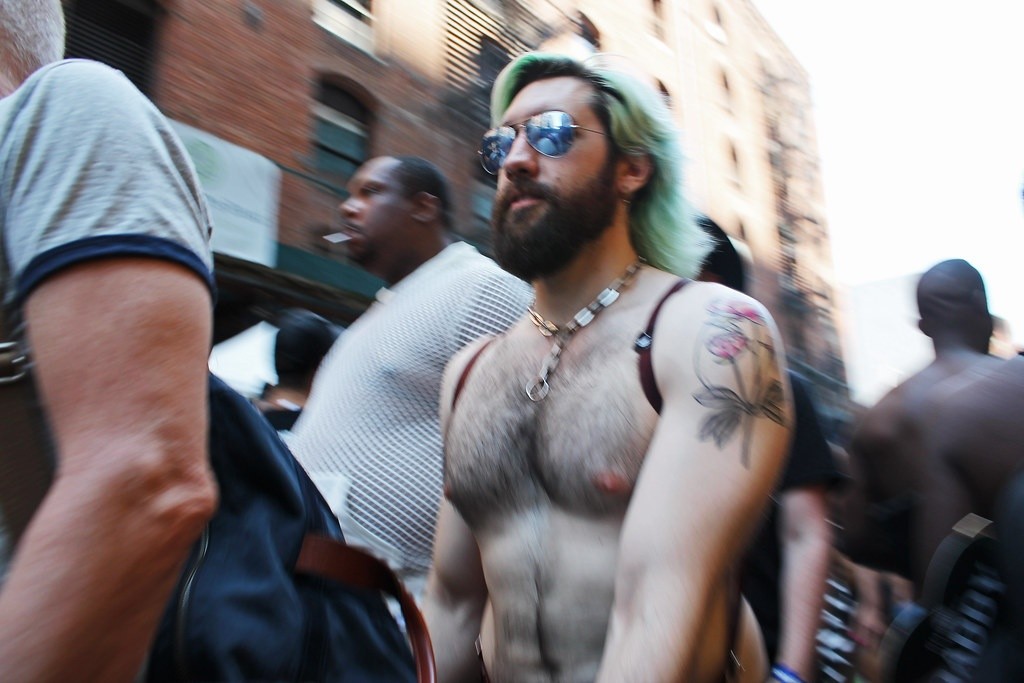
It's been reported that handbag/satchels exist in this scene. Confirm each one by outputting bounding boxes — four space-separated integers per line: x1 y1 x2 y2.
1 303 438 683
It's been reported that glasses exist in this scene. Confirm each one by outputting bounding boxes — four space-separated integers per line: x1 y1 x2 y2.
476 108 614 176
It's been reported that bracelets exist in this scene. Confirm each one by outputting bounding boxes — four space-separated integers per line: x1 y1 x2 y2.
771 662 803 683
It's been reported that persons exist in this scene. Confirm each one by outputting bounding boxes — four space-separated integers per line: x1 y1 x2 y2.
693 209 1023 683
420 48 794 682
0 0 536 683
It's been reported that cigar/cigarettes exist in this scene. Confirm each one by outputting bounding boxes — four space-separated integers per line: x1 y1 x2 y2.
323 233 349 243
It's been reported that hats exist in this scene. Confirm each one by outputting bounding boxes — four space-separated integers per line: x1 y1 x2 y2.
697 217 752 292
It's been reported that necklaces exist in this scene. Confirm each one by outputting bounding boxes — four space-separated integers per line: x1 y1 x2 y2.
525 257 644 400
273 396 300 410
